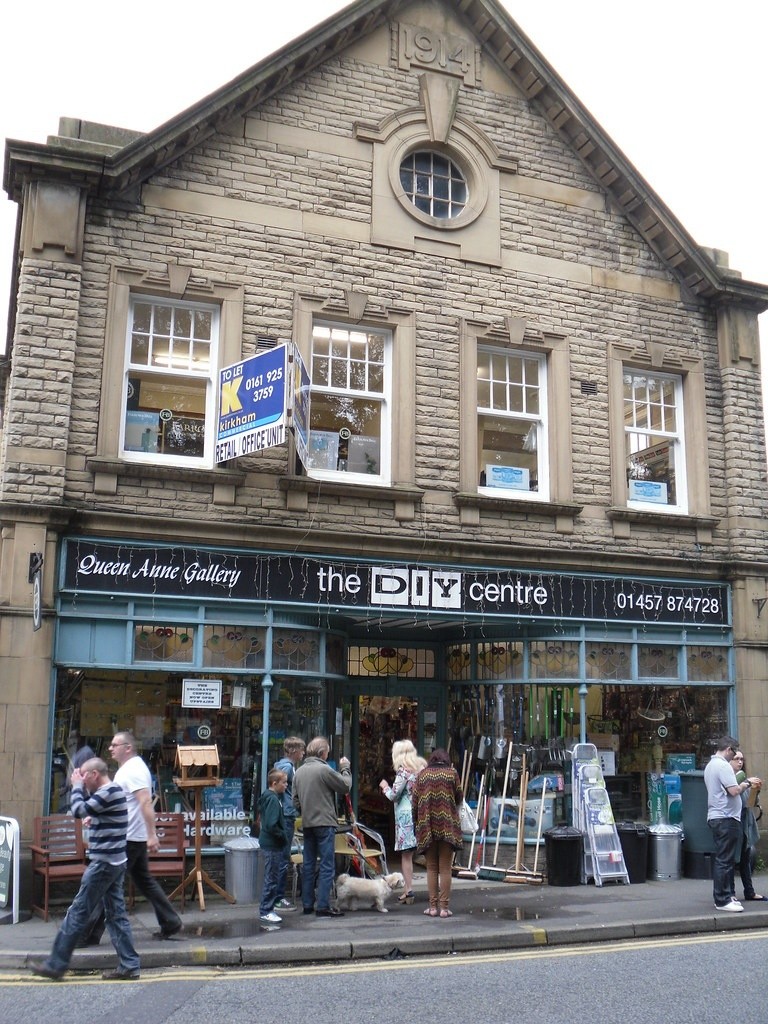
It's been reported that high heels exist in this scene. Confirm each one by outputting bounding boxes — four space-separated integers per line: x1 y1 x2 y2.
397 890 414 904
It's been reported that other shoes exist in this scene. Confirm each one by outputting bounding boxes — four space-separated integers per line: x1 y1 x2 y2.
153 923 184 939
439 910 453 918
731 896 737 901
745 895 768 901
74 937 98 948
424 909 438 916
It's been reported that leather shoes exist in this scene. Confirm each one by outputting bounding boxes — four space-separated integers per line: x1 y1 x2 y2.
102 969 139 980
303 908 314 914
28 961 62 981
316 909 345 917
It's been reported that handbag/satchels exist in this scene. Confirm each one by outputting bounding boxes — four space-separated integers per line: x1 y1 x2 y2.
458 798 479 835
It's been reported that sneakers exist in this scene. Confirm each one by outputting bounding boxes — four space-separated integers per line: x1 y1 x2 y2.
260 911 282 923
273 898 297 912
714 898 744 912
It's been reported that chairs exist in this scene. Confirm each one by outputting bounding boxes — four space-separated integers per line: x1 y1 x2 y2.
27 814 185 923
290 821 391 906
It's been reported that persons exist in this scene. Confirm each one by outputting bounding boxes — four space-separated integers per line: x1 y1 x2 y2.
273 735 305 911
379 739 428 904
84 732 181 945
60 728 97 812
703 735 768 912
291 736 353 917
411 748 464 917
255 769 290 922
29 757 142 980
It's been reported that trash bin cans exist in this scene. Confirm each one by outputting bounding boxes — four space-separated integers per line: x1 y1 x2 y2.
543 827 583 886
647 825 685 882
616 823 649 884
222 834 260 904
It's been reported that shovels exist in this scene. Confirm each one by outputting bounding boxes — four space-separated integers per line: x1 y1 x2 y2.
477 685 507 760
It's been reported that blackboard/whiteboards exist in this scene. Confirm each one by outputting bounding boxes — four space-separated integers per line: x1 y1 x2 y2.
203 780 243 816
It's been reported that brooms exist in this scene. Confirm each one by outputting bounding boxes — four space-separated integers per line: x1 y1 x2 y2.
503 753 544 885
456 774 484 880
476 741 513 882
451 750 471 878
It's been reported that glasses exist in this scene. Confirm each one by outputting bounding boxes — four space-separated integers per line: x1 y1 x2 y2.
112 743 129 748
731 748 737 756
731 757 743 760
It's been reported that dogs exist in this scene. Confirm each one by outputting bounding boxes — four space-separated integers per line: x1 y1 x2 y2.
333 872 405 912
